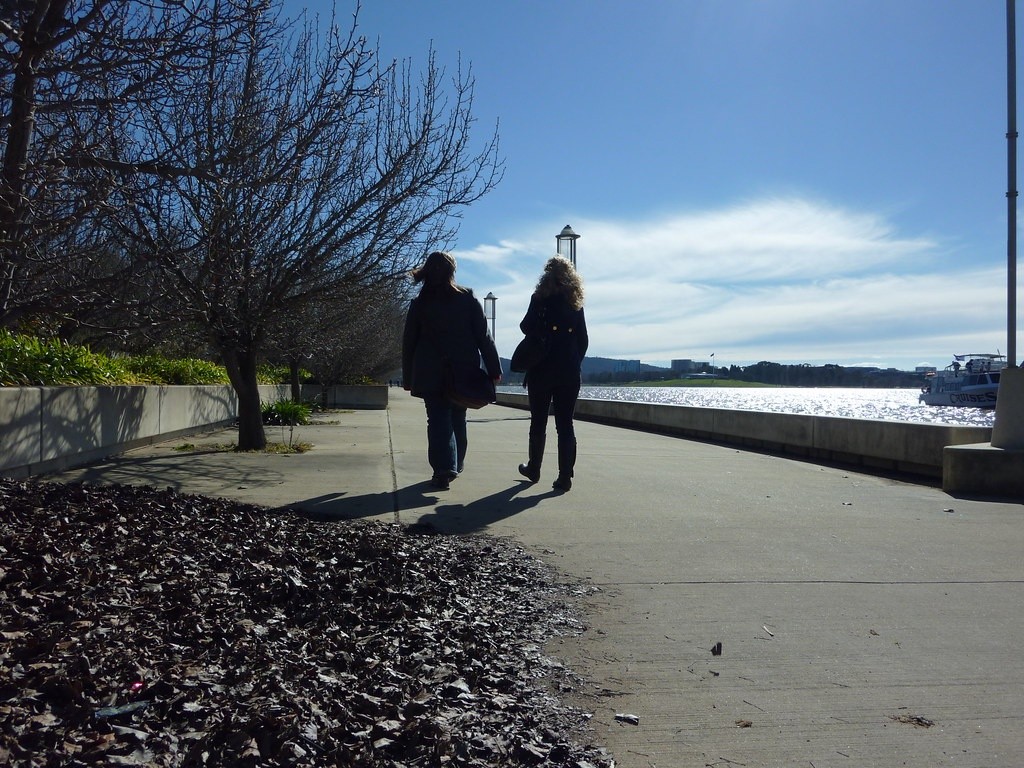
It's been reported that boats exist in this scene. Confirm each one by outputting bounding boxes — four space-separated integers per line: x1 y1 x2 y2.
918 347 1007 407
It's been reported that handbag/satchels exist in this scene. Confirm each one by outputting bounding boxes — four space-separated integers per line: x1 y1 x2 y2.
510 292 543 373
435 360 497 409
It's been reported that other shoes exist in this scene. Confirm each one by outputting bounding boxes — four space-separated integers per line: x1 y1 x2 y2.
430 475 451 492
552 478 572 491
519 464 540 483
457 468 463 473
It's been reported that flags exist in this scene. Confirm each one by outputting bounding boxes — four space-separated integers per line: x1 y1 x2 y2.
710 354 713 356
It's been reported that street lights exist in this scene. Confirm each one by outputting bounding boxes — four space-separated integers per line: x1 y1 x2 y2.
483 292 498 341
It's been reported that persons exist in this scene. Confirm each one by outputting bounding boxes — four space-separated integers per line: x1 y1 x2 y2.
966 359 991 373
518 257 588 490
403 251 503 488
952 361 961 377
